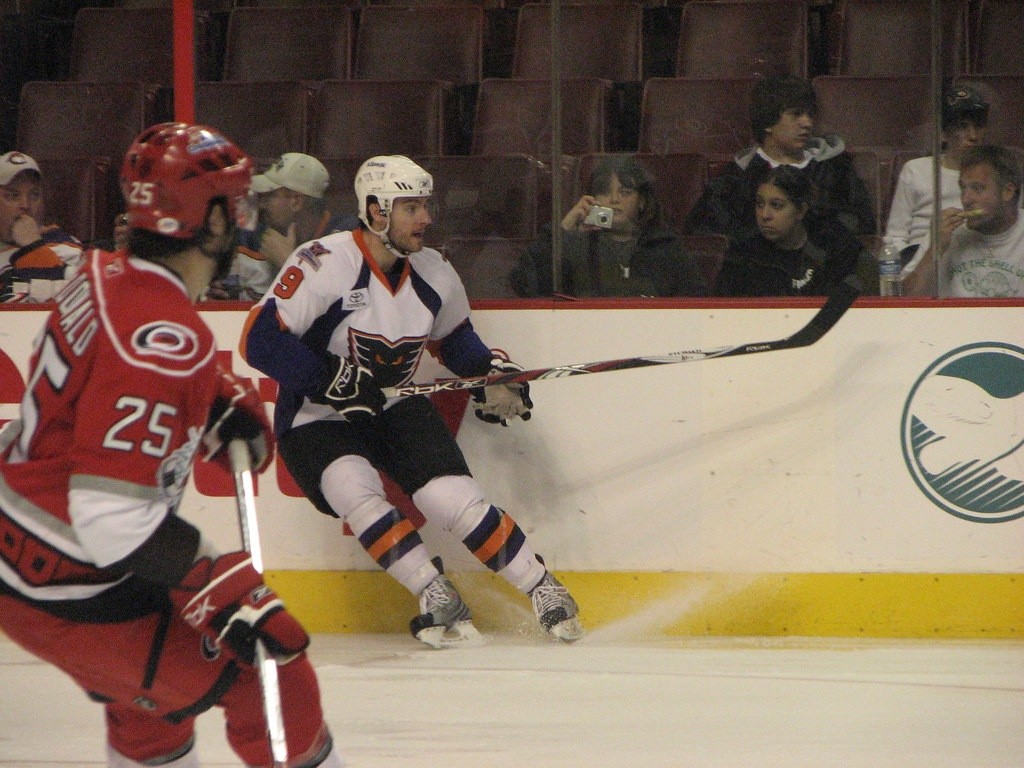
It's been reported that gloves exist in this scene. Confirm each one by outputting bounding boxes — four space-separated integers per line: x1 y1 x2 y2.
305 350 386 422
468 360 533 427
199 393 269 477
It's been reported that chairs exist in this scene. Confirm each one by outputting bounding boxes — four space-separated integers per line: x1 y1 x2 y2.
15 0 1024 302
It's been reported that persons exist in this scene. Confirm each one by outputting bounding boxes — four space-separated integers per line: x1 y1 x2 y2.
507 152 694 298
715 163 881 299
0 122 343 768
241 153 582 650
887 84 1024 266
683 69 876 256
204 153 345 302
899 143 1024 298
0 150 93 303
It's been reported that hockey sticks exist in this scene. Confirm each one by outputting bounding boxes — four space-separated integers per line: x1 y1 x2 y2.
226 432 291 767
385 273 863 399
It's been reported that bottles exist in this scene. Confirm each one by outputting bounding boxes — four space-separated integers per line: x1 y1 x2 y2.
879 236 902 296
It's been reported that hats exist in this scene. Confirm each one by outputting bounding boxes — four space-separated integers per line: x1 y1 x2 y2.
940 85 990 119
248 152 332 199
1 149 46 186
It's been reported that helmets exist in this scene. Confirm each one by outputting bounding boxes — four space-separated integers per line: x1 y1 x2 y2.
354 154 434 233
123 122 255 241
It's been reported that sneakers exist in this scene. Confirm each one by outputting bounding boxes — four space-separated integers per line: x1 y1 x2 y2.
408 575 486 650
529 573 584 643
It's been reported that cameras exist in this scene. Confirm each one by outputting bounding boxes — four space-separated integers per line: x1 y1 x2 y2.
582 206 613 228
